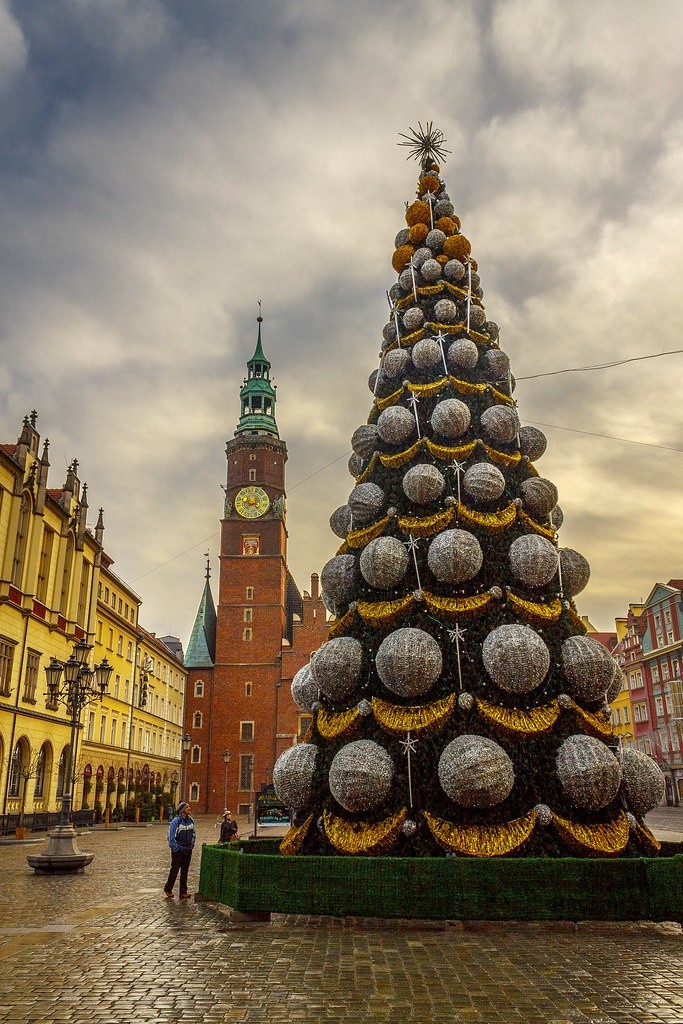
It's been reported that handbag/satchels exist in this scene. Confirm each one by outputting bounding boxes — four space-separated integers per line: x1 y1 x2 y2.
230 832 240 841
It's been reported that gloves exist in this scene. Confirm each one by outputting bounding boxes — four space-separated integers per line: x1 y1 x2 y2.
231 828 236 833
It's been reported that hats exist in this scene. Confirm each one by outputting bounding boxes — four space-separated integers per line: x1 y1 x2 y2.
223 811 232 817
177 802 188 813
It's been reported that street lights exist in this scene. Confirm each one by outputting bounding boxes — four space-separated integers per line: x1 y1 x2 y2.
26 638 115 877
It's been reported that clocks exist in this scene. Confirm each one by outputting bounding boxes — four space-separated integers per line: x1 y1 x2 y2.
282 494 286 521
234 487 269 519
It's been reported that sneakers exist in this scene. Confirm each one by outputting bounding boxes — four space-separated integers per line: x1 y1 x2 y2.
179 892 192 898
166 891 174 897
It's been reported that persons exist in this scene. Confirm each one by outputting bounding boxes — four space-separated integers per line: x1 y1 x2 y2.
164 801 196 898
167 804 172 822
217 810 238 844
